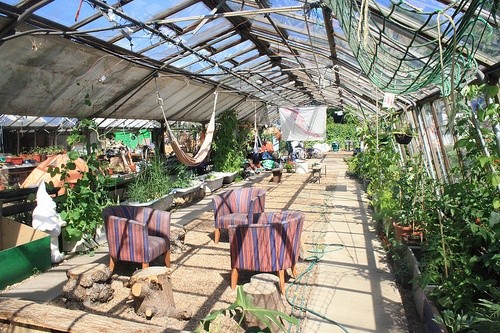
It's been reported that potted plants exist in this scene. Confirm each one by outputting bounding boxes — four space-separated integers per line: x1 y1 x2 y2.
393 111 416 144
392 154 430 244
197 171 224 193
171 163 207 209
44 116 115 252
6 145 67 165
213 158 244 183
121 147 174 210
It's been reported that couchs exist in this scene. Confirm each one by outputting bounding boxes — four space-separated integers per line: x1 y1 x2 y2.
212 187 265 243
227 211 306 295
102 204 171 272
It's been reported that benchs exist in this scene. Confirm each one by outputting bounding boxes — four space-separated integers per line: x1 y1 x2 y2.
312 163 329 184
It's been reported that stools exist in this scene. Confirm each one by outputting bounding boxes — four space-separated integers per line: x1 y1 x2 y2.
272 167 283 182
